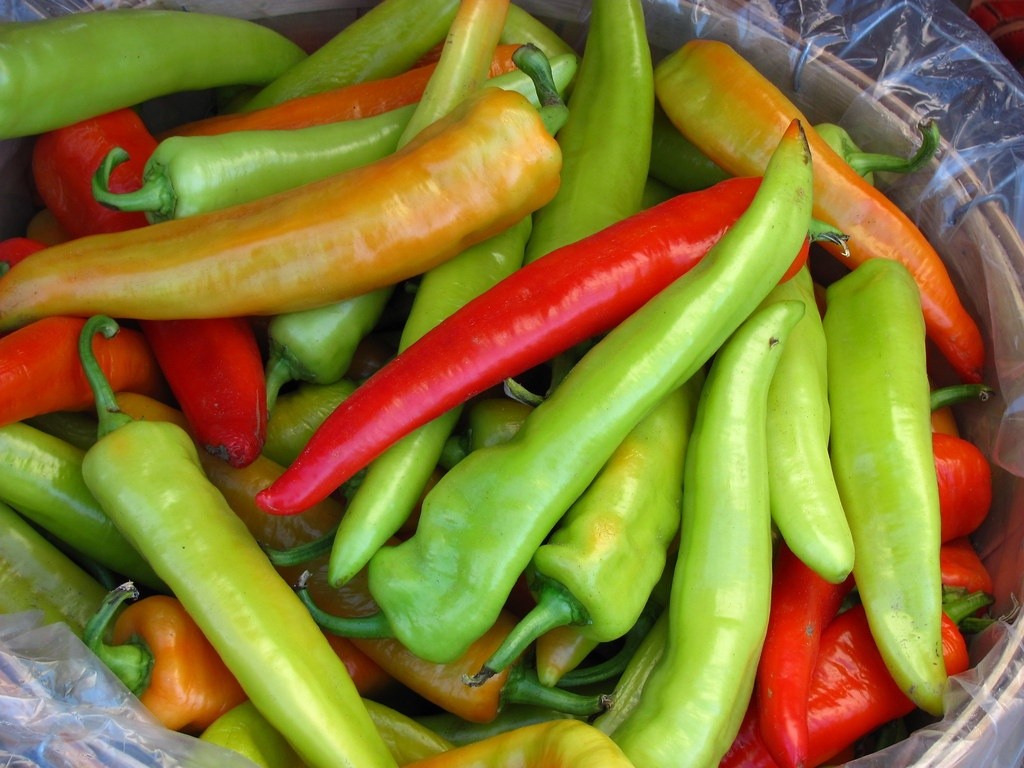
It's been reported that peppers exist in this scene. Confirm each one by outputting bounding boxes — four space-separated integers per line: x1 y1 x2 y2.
0 0 1020 768
